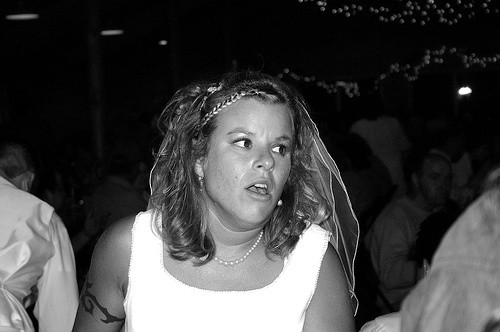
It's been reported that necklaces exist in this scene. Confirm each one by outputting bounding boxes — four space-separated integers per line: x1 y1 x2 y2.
206 229 264 265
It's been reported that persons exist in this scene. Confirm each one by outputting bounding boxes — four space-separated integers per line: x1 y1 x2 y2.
331 86 500 332
71 67 359 331
0 108 156 332
